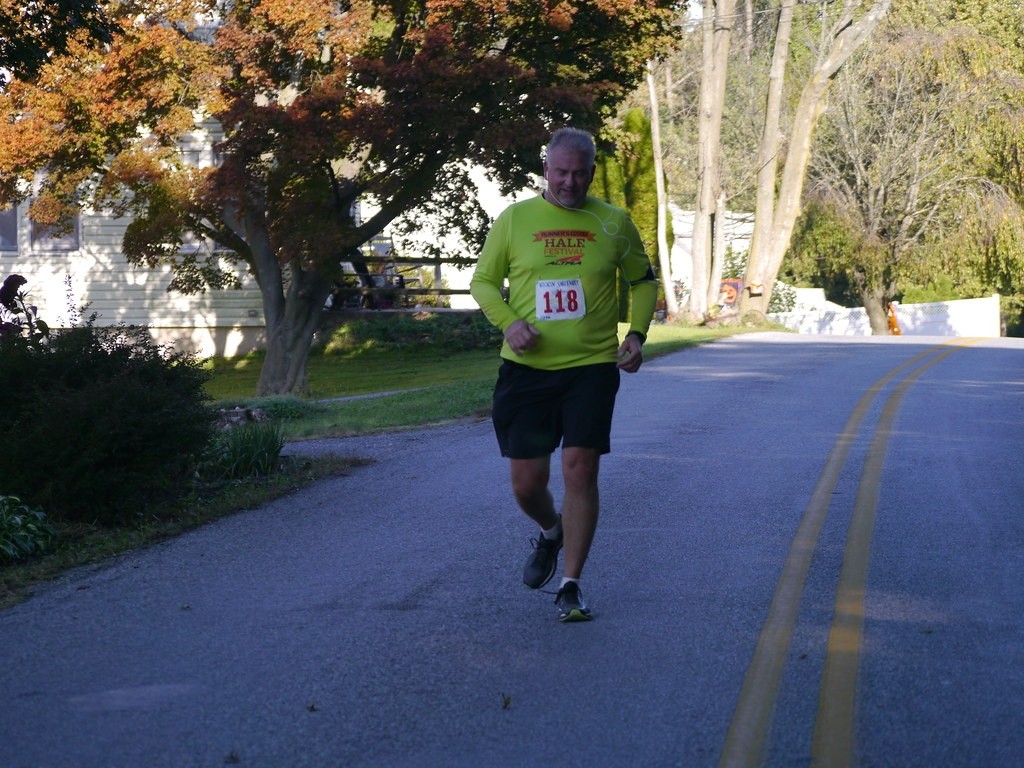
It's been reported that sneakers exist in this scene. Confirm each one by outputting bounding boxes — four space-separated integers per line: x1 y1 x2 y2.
522 512 564 588
543 581 592 621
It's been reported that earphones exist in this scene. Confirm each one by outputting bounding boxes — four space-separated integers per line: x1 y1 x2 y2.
546 171 550 179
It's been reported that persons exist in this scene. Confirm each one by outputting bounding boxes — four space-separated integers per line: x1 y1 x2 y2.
470 127 658 621
